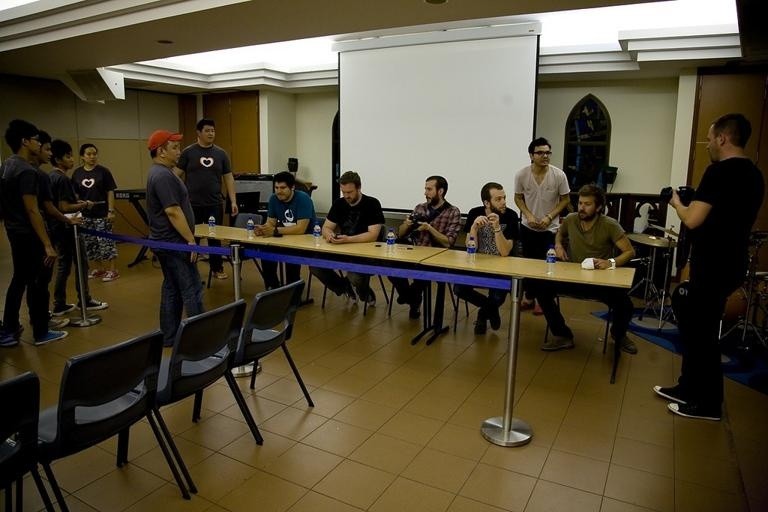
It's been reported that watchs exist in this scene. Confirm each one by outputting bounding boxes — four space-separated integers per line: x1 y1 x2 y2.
274 226 278 236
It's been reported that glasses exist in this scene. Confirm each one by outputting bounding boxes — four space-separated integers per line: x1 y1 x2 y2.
531 151 552 157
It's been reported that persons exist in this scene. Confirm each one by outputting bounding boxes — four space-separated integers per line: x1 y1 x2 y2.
387 176 462 317
309 171 385 304
146 130 204 345
175 120 239 279
74 144 120 282
26 132 71 330
514 137 570 314
453 183 520 334
0 119 69 347
653 113 765 422
537 187 636 354
254 171 316 289
45 140 109 317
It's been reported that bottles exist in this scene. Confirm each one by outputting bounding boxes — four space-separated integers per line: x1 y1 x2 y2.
386 227 396 255
247 217 255 241
208 214 216 233
545 244 558 277
312 221 322 246
466 235 477 264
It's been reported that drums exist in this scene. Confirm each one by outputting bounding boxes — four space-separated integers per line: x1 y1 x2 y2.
672 280 747 336
742 270 768 332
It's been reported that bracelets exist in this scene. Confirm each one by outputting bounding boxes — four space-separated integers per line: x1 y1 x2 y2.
608 258 615 268
107 210 114 212
495 226 501 232
546 214 553 222
86 201 88 212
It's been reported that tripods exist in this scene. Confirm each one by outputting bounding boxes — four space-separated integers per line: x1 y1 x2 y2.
628 246 661 307
718 241 768 352
638 234 677 331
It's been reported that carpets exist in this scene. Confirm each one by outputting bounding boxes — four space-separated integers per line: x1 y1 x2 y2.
590 305 768 396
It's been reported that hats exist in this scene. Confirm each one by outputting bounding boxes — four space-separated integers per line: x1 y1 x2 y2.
146 128 185 152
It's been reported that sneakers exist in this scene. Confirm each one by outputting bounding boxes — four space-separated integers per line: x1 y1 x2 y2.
343 276 359 305
651 382 690 405
76 298 109 310
610 331 637 354
51 303 76 316
33 330 70 347
394 289 502 337
88 268 119 282
366 286 377 307
539 328 576 351
46 317 72 331
519 299 546 316
211 271 228 279
665 398 724 420
0 319 24 350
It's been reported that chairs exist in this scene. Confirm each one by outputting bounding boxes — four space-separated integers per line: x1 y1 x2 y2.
207 213 265 289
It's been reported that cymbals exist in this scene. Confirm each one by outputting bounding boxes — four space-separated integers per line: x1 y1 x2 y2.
651 224 679 237
749 230 768 246
627 233 677 247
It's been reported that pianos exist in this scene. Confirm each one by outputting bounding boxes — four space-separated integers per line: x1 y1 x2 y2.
114 188 146 201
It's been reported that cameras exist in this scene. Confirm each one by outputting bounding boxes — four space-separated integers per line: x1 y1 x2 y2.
408 212 424 225
660 186 696 207
